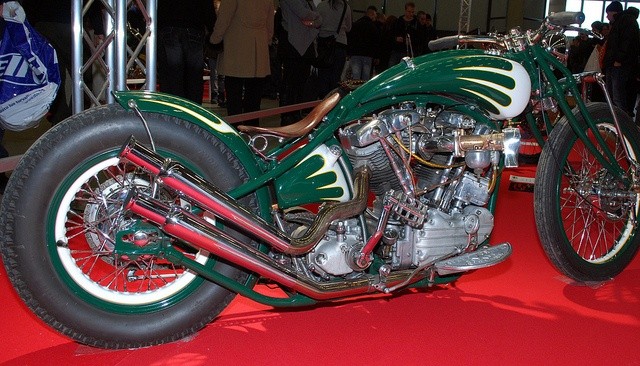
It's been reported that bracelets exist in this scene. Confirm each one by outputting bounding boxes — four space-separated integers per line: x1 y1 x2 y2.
310 21 313 26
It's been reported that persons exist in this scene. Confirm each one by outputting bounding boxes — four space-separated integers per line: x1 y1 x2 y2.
19 0 105 124
276 0 324 126
312 0 352 88
567 20 609 103
138 0 224 105
341 2 434 83
210 0 275 130
601 1 640 113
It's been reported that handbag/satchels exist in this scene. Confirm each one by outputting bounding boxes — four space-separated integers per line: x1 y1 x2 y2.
287 36 347 103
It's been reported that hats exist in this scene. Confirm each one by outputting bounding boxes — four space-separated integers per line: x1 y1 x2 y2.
605 0 623 14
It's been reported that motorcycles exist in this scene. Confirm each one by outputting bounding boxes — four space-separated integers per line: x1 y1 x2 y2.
0 11 640 348
